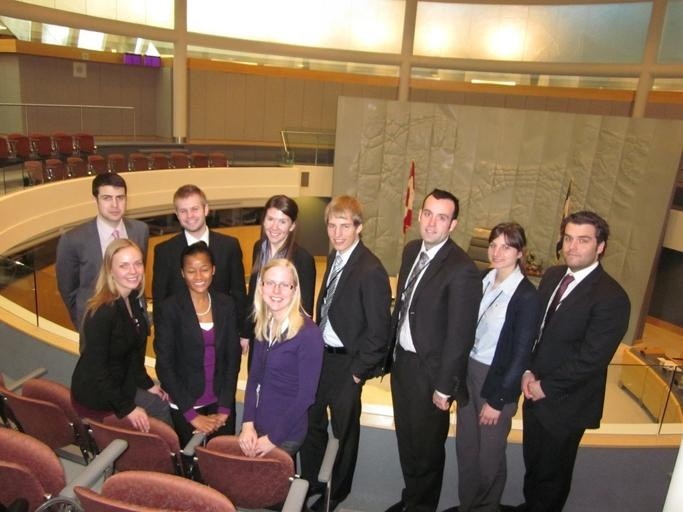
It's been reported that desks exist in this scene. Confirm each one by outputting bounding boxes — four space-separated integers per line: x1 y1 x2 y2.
617 345 683 423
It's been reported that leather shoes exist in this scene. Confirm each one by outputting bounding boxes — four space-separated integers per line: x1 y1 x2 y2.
384 500 408 512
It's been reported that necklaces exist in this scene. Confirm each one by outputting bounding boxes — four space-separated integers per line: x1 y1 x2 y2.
190 291 210 317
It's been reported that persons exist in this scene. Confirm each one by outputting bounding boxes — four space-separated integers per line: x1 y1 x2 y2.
518 208 630 512
451 222 541 512
151 239 243 446
148 182 246 345
68 236 169 437
239 193 317 377
385 188 482 512
296 194 393 512
235 255 323 464
53 171 148 332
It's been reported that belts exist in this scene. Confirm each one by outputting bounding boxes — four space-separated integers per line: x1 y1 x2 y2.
324 344 349 355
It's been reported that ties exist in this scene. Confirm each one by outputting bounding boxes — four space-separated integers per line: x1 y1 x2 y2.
394 253 431 332
318 256 345 336
112 231 120 241
542 272 576 331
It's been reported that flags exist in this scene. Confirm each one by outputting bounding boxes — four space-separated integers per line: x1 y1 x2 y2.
554 178 573 260
402 161 414 234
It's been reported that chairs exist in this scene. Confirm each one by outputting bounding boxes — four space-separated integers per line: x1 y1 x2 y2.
466 228 491 270
0 131 230 187
